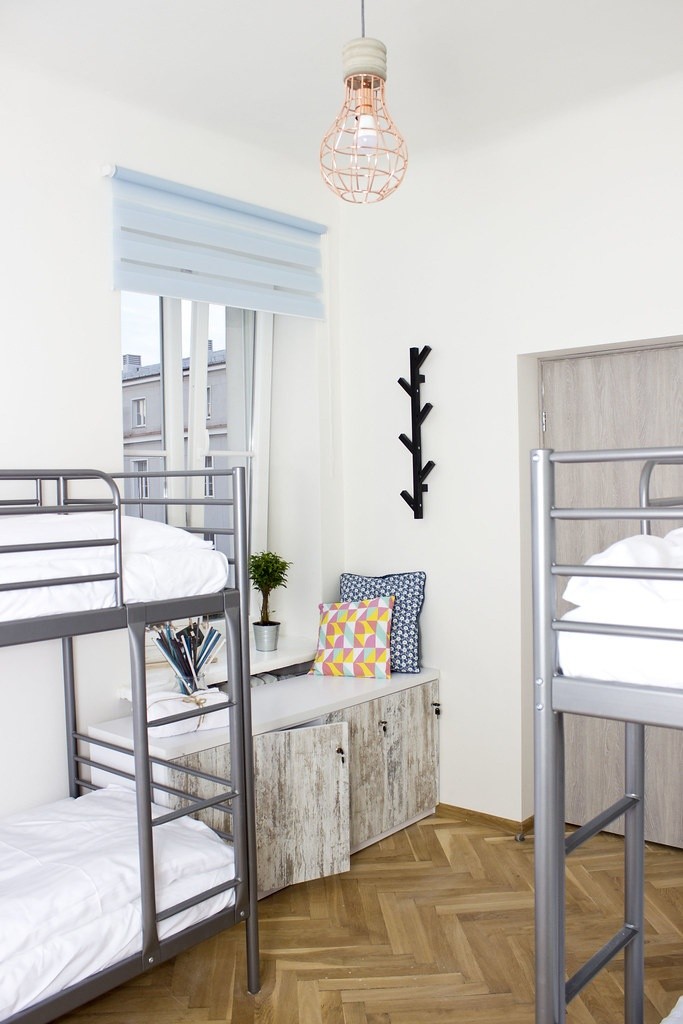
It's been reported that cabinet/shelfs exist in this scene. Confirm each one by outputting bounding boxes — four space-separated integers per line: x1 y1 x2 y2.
253 715 350 899
165 740 252 913
382 680 440 832
325 696 382 856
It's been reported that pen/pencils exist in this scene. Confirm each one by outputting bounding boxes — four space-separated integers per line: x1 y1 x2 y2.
153 625 226 693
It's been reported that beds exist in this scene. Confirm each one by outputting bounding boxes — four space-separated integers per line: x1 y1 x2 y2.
0 459 247 646
0 735 259 1024
530 446 683 731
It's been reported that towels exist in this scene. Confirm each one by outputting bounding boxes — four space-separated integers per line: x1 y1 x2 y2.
147 687 229 738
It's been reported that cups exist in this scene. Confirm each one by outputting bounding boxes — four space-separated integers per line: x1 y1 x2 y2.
176 673 205 696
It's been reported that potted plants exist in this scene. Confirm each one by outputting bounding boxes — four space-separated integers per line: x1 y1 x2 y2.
247 550 293 652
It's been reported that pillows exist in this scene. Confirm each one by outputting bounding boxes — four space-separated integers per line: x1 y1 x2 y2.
307 596 394 680
340 571 426 674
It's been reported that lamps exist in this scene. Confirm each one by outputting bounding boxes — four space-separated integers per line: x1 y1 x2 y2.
320 0 410 203
